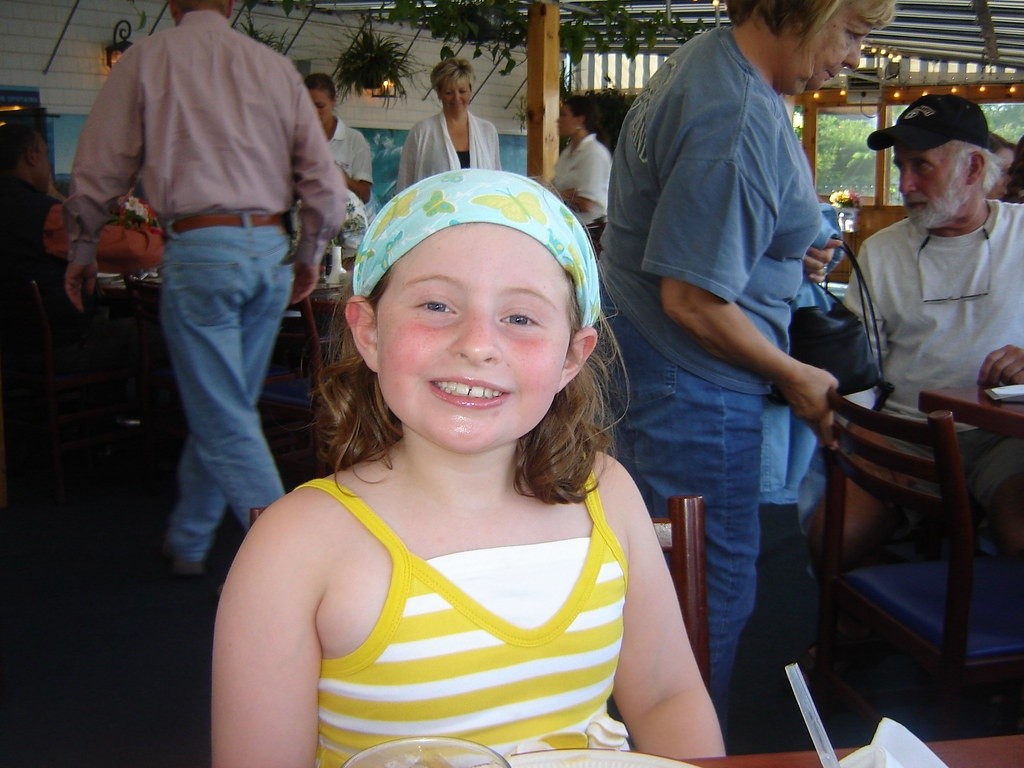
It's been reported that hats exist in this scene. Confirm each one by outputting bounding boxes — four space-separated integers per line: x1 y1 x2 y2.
866 94 988 150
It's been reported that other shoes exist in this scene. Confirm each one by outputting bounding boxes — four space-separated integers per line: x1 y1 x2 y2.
114 409 142 426
160 535 206 575
799 624 875 670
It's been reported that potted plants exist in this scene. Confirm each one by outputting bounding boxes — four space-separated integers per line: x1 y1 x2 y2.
295 2 439 111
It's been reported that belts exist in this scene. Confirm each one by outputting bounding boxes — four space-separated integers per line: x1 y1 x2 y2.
162 216 283 241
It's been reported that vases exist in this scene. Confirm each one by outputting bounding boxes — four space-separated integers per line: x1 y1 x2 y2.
837 208 859 233
330 247 347 283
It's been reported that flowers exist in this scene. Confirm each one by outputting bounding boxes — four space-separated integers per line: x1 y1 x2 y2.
829 190 860 208
107 195 160 230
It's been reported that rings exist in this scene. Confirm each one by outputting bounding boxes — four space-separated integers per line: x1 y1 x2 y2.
824 266 826 273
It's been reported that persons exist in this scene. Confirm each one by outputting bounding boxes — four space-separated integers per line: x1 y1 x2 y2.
598 1 897 754
397 58 502 195
66 0 346 582
799 92 1024 676
986 133 1024 204
550 95 613 257
211 169 726 768
0 123 166 481
291 73 374 205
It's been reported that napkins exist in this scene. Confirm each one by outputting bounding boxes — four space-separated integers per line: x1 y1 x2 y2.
838 717 950 768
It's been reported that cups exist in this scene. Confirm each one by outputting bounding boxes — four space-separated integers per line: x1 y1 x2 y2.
337 736 513 768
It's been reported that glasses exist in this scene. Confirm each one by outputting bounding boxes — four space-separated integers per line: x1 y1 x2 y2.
564 94 577 104
917 228 992 303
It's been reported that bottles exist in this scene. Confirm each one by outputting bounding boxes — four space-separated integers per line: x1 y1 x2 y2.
326 246 347 284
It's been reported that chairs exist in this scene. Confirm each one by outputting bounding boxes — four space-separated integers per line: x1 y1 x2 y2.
25 275 336 483
816 388 1024 740
653 493 712 691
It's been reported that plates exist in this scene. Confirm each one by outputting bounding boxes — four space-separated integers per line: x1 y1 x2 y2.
471 748 702 768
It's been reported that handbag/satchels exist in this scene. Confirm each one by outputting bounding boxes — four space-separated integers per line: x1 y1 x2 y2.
790 300 879 396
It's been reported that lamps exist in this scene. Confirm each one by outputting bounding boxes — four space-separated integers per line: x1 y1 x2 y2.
105 20 133 71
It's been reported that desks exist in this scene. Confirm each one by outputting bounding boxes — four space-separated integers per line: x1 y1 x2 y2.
817 202 844 292
917 389 1024 441
88 280 339 315
